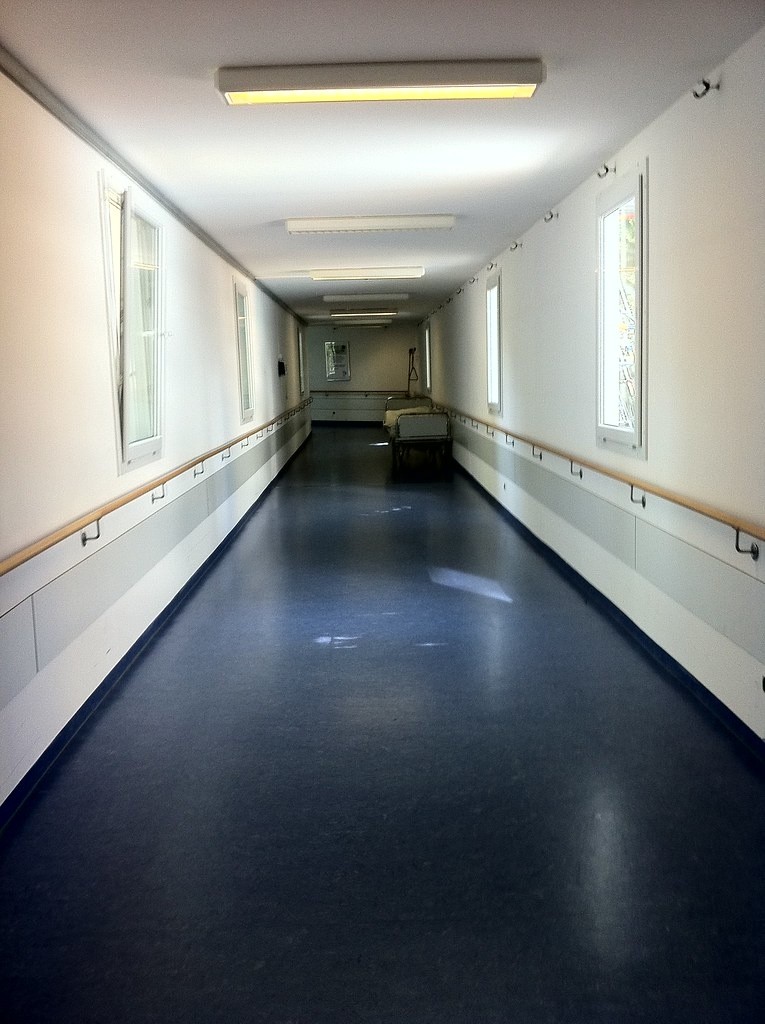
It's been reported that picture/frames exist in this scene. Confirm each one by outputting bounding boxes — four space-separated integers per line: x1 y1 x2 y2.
324 341 351 381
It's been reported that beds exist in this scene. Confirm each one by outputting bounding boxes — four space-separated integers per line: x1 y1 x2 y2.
384 396 453 472
